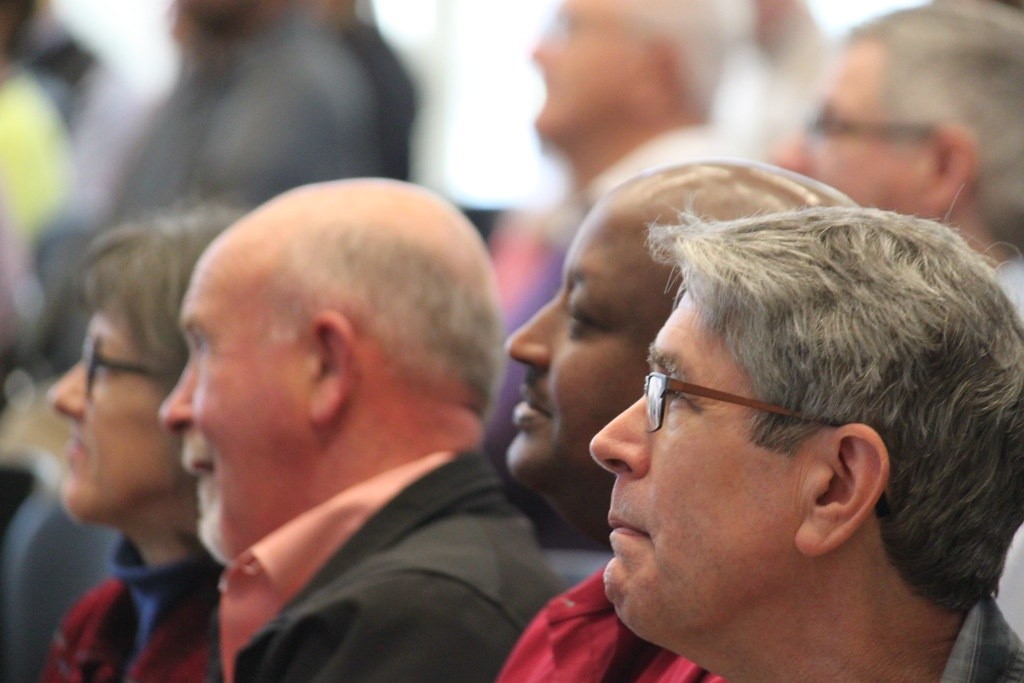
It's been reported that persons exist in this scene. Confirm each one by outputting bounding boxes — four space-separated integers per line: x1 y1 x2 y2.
590 207 1024 682
779 0 1024 262
488 0 754 466
155 176 571 682
41 203 243 683
1 1 422 377
494 162 862 683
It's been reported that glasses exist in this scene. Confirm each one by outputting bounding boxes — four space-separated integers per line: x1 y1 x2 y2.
799 92 945 160
76 334 177 400
641 371 846 434
